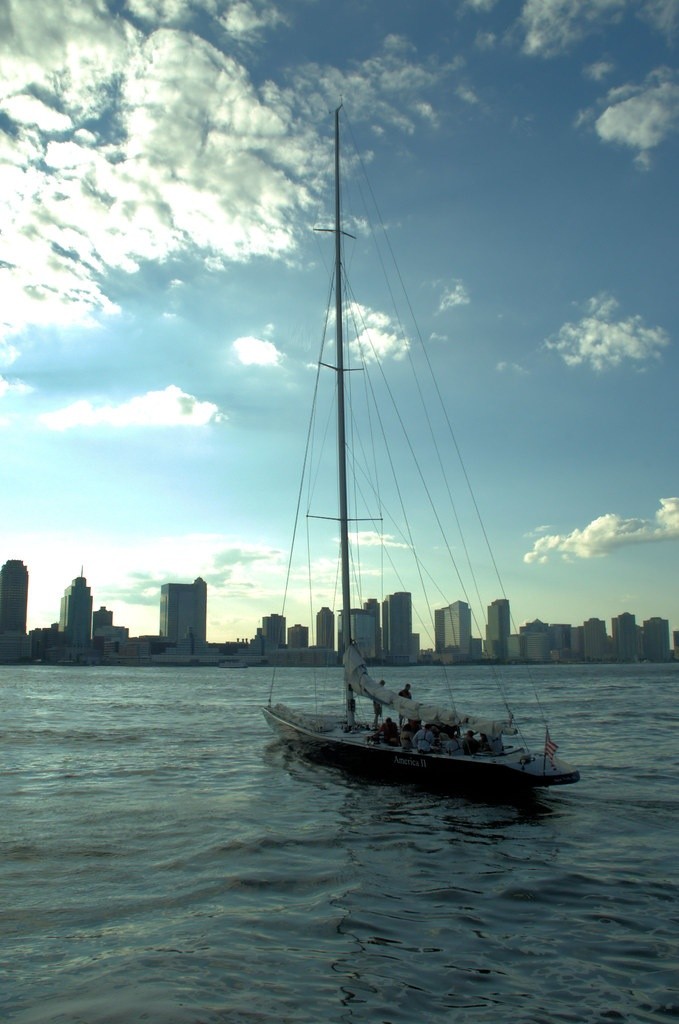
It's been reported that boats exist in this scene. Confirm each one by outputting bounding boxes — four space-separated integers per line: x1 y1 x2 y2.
219 661 248 669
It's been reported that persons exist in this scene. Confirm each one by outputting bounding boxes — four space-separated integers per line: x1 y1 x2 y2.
373 679 505 755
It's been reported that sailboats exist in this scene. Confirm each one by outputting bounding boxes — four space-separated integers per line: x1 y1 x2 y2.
263 105 583 789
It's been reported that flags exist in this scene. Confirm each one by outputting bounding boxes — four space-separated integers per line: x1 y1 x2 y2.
545 729 558 768
